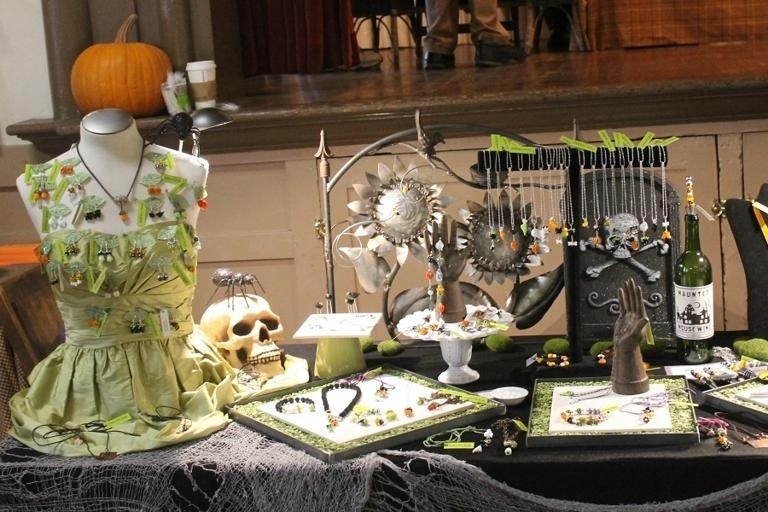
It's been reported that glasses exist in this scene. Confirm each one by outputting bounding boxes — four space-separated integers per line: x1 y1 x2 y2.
147 109 233 151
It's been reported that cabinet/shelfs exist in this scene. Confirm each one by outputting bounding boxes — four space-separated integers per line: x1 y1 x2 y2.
674 214 715 363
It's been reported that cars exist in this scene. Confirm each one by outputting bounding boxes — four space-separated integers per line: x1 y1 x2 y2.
160 60 217 117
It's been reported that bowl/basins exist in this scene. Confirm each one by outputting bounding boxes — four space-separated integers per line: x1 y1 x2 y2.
76 139 145 221
559 386 611 404
621 391 667 423
373 374 396 399
322 382 360 431
426 237 446 313
697 410 767 450
423 424 494 453
483 146 671 255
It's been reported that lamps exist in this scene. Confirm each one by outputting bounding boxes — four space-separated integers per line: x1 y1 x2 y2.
316 118 584 314
1 263 64 386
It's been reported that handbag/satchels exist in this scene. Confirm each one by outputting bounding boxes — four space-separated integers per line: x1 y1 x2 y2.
275 397 316 414
460 310 484 332
412 316 449 337
535 353 571 367
561 406 604 428
496 415 525 456
354 406 396 427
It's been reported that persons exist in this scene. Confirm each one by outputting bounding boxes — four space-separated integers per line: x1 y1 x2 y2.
7 109 237 458
613 278 650 394
425 214 475 323
421 0 519 70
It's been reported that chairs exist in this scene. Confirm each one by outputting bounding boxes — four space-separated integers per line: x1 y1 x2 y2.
424 50 455 69
475 39 526 67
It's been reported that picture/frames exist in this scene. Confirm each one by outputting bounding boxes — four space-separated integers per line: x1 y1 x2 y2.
0 330 768 511
349 0 520 58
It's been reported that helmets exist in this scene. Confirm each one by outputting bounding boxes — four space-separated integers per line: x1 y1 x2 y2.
490 386 529 406
